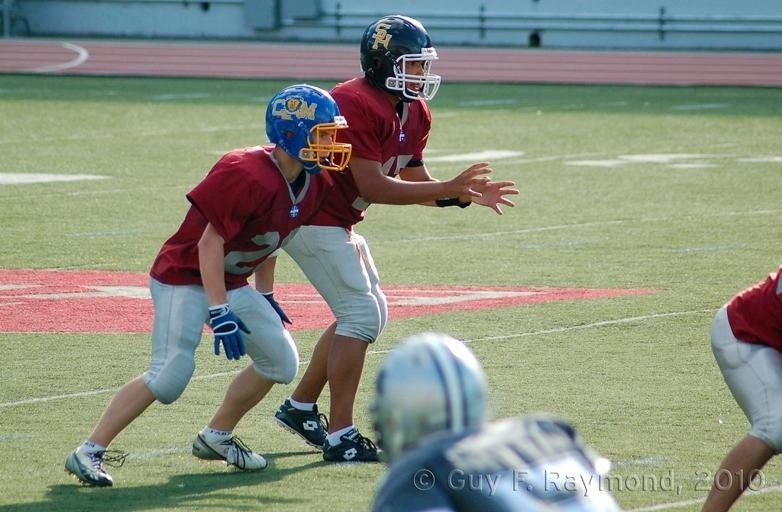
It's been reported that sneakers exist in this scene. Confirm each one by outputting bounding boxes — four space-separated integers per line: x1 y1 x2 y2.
275 398 329 449
193 431 267 469
64 447 129 486
323 427 384 460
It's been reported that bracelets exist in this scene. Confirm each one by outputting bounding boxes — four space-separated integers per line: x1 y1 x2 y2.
435 197 472 208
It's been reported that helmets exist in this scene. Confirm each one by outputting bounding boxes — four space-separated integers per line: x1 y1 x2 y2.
370 332 489 460
361 15 441 102
266 84 353 174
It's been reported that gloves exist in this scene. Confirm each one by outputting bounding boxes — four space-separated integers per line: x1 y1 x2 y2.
208 303 251 359
261 291 293 328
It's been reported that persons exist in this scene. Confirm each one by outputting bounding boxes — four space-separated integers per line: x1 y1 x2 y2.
368 331 622 512
65 84 353 487
701 267 782 512
275 15 519 458
